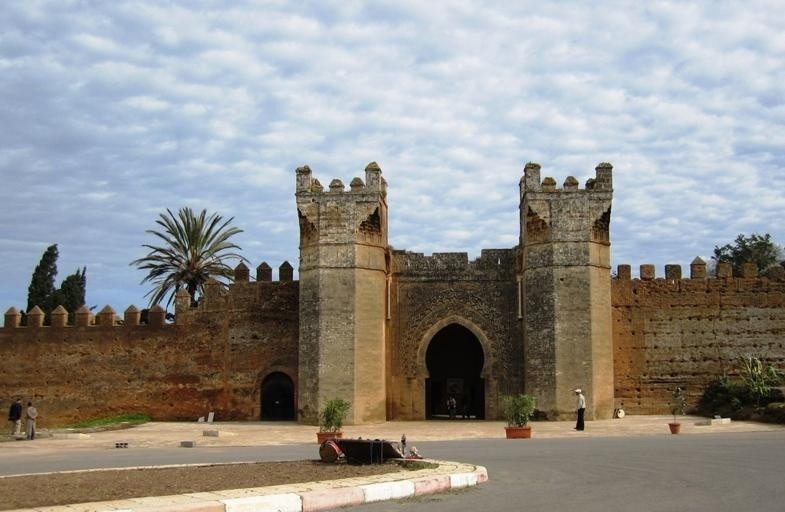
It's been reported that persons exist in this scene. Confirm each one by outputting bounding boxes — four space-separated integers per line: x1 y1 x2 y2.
8 398 22 436
25 400 38 440
444 392 471 420
573 388 586 431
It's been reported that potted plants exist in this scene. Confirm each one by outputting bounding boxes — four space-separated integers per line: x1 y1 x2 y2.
504 393 537 439
316 397 353 444
668 386 687 434
